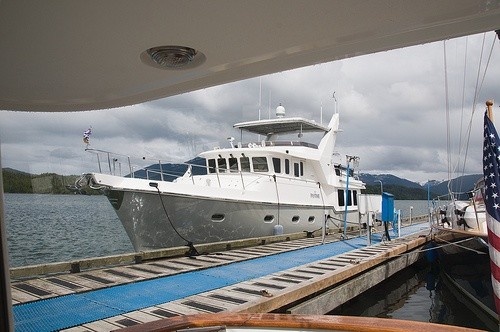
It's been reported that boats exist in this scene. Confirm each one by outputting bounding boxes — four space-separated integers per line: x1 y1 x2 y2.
74 74 383 252
446 175 488 233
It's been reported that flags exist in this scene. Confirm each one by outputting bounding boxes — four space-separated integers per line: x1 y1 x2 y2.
483 109 500 314
